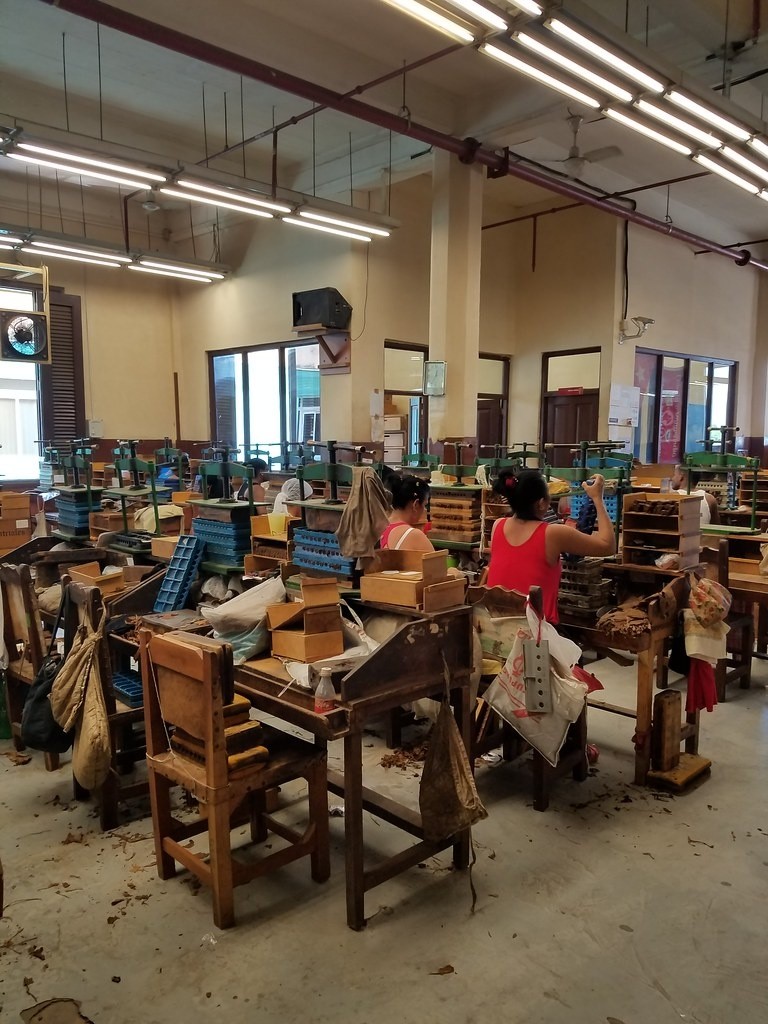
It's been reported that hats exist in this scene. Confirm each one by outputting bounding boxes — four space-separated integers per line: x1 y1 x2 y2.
171 451 189 469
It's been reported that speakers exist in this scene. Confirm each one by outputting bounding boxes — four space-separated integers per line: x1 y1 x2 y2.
292 287 354 330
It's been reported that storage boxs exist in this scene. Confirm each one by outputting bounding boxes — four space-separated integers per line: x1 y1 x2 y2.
0 413 768 776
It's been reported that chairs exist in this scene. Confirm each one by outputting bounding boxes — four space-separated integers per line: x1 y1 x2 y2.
136 627 332 932
61 575 199 833
655 539 754 702
460 572 588 812
0 561 60 772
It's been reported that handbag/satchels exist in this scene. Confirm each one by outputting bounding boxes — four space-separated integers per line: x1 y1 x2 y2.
20 586 75 752
71 607 112 791
480 627 589 767
690 570 733 630
419 650 489 845
47 598 106 734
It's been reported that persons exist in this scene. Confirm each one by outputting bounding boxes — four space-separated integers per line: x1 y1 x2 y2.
272 478 314 517
671 464 721 525
380 474 436 551
487 468 616 770
148 451 236 498
238 458 269 515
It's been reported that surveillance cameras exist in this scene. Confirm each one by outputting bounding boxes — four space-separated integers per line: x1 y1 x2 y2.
637 317 655 325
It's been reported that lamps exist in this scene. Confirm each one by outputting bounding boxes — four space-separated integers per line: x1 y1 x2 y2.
0 0 768 285
